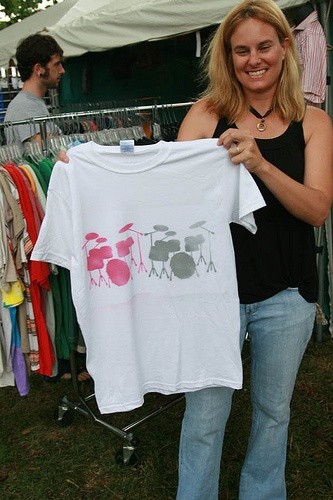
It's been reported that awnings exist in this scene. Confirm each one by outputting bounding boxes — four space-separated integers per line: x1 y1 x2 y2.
0 1 333 68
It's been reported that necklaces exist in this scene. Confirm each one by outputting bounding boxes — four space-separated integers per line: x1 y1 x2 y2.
248 105 273 131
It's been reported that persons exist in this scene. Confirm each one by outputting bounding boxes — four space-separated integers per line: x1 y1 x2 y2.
58 1 333 500
3 34 66 143
